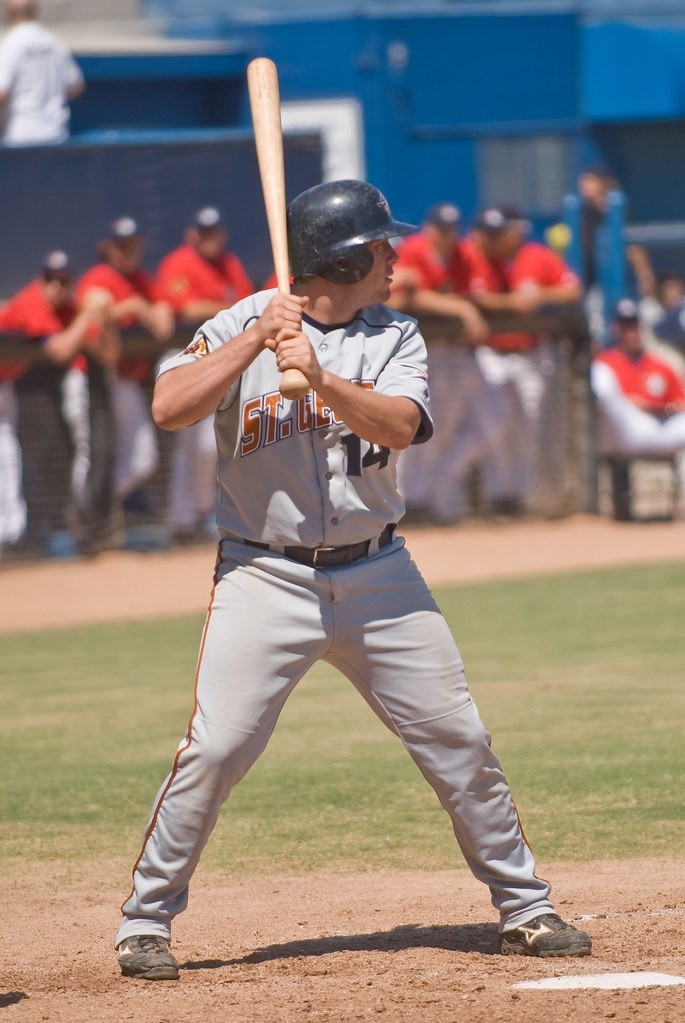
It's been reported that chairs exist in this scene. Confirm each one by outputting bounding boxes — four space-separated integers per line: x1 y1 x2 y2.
572 356 679 521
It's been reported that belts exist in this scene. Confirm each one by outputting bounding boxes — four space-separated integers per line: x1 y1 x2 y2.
243 524 393 570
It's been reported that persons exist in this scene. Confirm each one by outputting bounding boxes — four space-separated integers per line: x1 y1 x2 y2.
110 179 590 981
566 170 685 520
159 206 256 541
0 247 111 558
386 200 583 527
62 221 172 559
0 0 86 152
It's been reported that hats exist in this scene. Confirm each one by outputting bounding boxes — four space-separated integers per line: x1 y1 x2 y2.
106 216 143 240
606 295 639 327
193 206 227 230
429 203 461 228
39 251 79 284
475 206 534 232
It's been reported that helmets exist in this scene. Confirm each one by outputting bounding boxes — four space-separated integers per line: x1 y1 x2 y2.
286 179 419 285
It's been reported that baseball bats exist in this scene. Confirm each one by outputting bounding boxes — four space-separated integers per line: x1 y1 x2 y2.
244 56 313 402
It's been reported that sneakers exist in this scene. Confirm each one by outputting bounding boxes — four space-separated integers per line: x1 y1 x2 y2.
499 914 592 957
117 935 179 979
